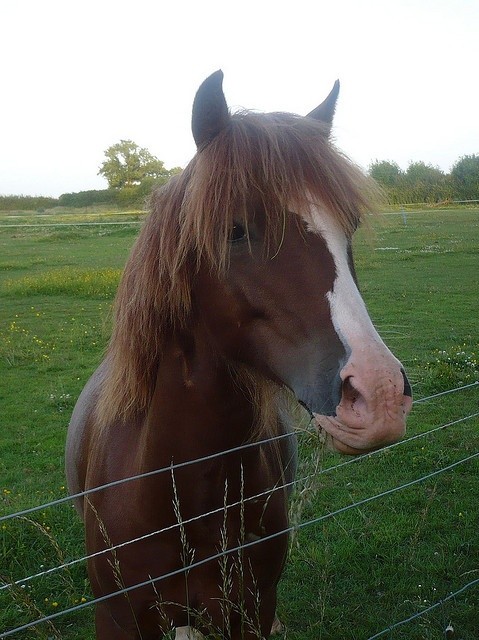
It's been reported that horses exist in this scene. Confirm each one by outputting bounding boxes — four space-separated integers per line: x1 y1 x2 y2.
61 67 415 640
